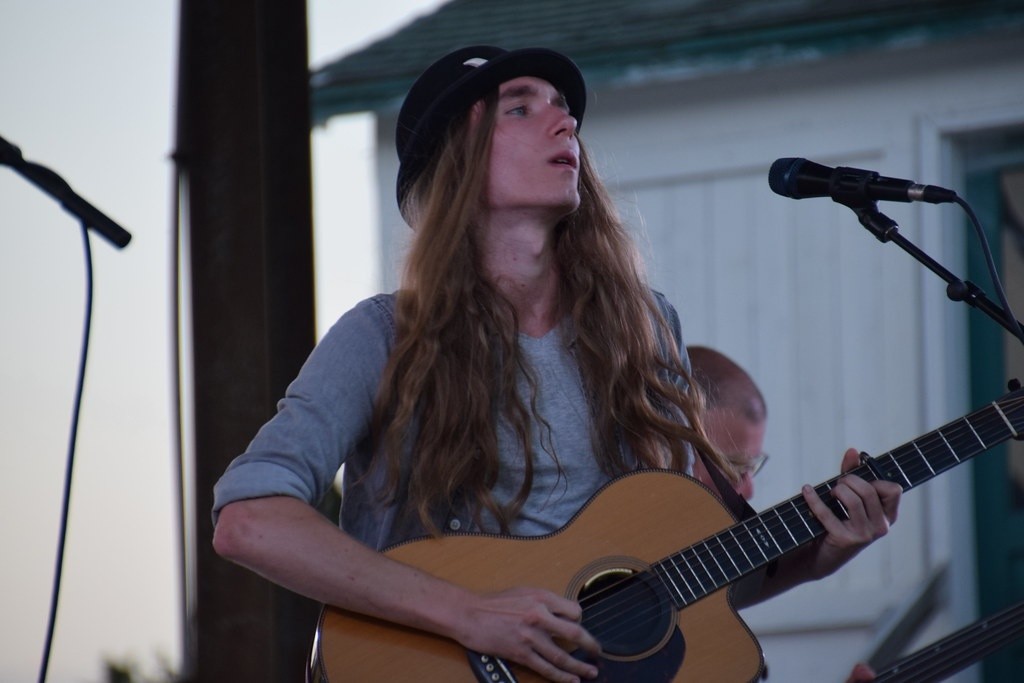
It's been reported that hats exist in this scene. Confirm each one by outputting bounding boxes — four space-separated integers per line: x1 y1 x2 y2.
396 44 587 234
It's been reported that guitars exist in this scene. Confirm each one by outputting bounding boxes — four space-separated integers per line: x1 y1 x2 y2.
866 599 1023 682
306 375 1024 682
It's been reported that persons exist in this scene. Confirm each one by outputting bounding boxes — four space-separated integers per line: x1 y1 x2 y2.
209 44 908 683
685 344 768 504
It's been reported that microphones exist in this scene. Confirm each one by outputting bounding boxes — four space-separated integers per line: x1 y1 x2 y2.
768 158 957 205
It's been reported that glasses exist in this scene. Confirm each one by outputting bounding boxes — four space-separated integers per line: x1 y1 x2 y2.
724 454 770 477
